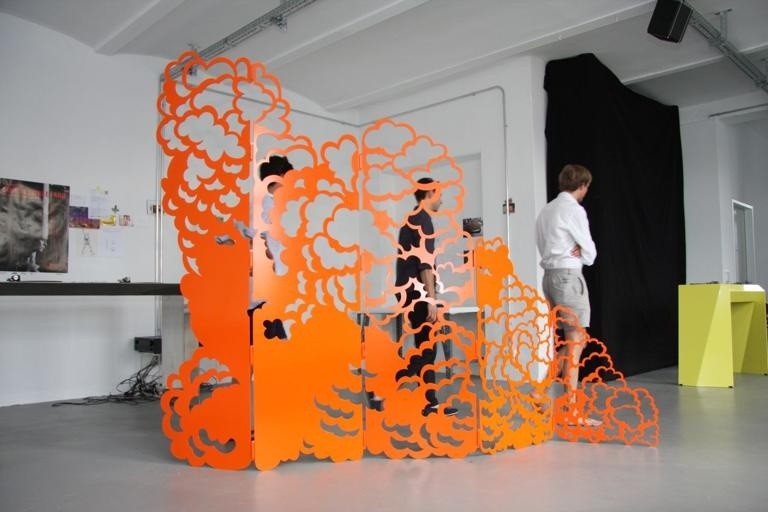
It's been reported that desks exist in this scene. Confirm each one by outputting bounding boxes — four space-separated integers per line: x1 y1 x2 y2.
677 282 768 390
368 307 483 379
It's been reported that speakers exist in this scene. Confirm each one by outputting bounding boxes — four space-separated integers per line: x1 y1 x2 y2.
646 0 692 44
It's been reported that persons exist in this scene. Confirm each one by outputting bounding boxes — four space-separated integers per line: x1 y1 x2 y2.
364 177 459 418
216 154 296 339
526 163 605 428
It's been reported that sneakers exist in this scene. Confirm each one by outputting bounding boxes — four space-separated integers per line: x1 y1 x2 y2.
527 389 549 413
423 406 458 416
363 392 381 411
556 417 602 426
